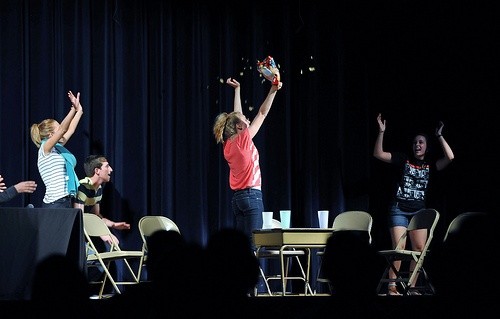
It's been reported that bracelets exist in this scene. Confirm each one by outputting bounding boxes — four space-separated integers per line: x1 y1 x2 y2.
380 129 384 132
437 134 443 137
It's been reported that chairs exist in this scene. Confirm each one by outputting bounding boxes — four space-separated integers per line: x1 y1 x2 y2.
377 208 440 296
81 212 144 299
137 216 180 283
314 211 373 294
422 211 479 296
254 246 314 296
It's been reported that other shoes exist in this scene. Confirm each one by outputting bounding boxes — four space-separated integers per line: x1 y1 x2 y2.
387 284 401 296
406 284 422 295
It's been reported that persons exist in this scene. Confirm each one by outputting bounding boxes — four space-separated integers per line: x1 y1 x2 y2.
72 155 130 253
374 114 454 296
27 211 500 319
0 175 37 206
31 91 83 209
213 77 283 252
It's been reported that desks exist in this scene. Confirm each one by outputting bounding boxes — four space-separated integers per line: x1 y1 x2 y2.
255 228 334 297
0 208 90 307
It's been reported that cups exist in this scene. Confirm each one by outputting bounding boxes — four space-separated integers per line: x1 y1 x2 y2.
280 210 291 229
318 211 329 228
262 212 273 229
